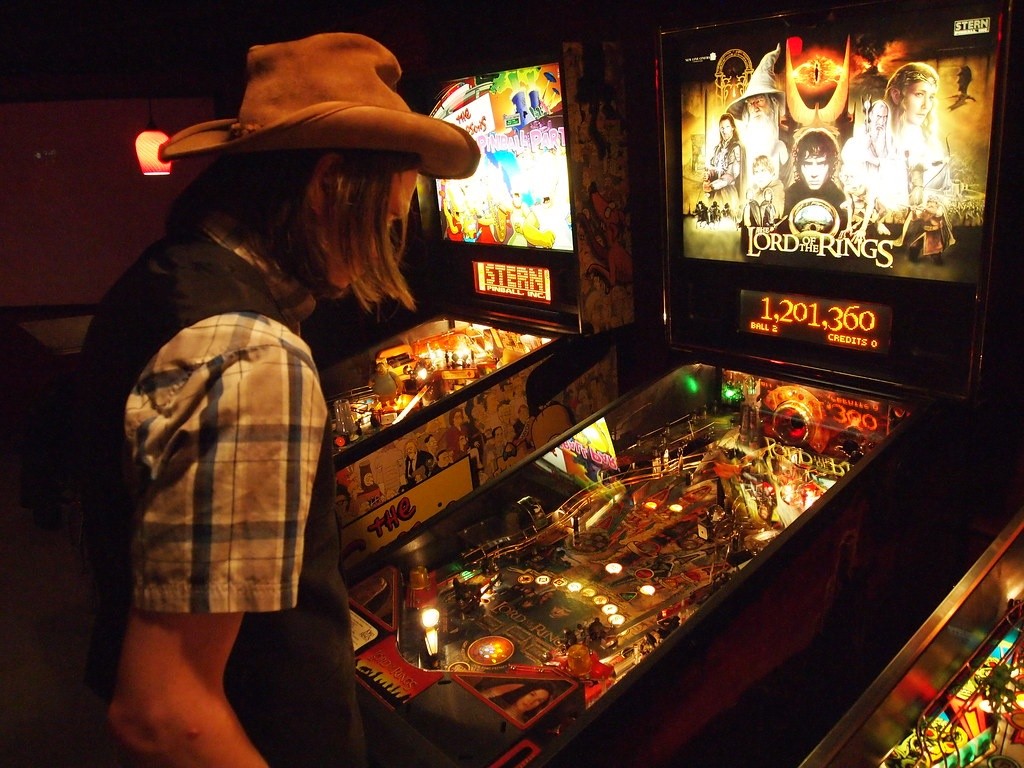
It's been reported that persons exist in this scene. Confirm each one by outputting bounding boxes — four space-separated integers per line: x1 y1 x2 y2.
697 43 961 238
4 28 477 768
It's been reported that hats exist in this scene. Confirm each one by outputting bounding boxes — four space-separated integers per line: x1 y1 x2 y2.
158 32 479 178
